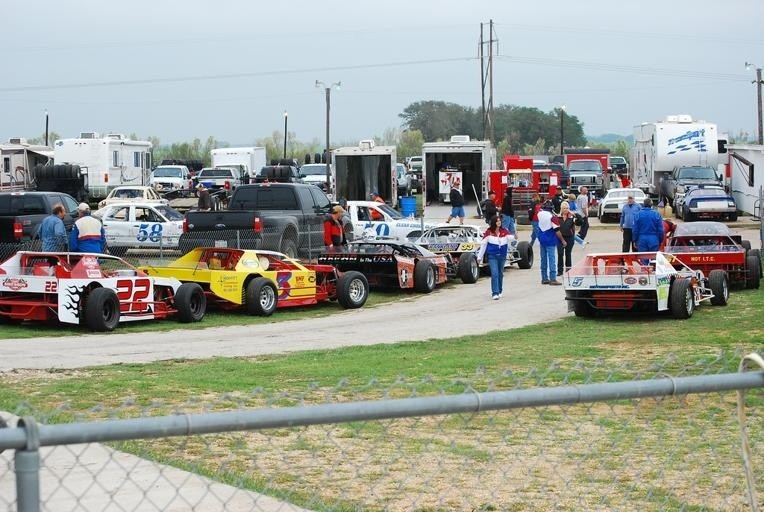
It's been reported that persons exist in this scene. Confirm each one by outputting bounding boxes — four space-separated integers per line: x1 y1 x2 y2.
324 192 353 254
659 220 676 252
633 198 663 266
252 170 257 183
187 172 212 212
244 171 249 184
369 192 385 220
322 149 327 163
445 181 465 225
477 216 518 300
69 202 110 265
483 190 502 224
38 203 68 252
498 186 519 239
528 186 590 286
660 172 677 217
619 195 642 252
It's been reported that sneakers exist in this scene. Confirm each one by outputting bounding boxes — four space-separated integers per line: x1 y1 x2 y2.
499 293 503 297
492 295 499 300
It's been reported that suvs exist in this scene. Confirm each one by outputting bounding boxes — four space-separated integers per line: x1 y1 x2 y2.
152 165 192 196
487 154 611 220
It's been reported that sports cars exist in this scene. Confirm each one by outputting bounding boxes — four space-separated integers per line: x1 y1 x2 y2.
560 251 729 318
662 222 762 288
0 249 207 331
415 226 534 283
318 239 453 293
596 188 652 222
140 246 369 317
675 185 737 221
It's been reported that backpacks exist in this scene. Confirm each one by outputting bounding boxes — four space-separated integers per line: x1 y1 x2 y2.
477 200 490 218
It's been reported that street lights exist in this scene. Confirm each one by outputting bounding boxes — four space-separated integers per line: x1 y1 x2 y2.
744 61 763 145
314 77 341 193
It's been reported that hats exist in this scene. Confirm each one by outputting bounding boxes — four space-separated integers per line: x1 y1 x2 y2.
77 203 91 212
195 183 203 188
369 191 379 196
331 205 344 214
488 190 496 195
644 199 654 205
556 185 562 189
454 183 460 185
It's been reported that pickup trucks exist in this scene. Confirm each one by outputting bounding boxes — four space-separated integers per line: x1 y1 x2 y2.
255 166 306 183
663 165 724 197
0 192 79 256
179 181 334 259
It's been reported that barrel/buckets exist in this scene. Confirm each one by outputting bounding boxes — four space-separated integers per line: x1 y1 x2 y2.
398 196 417 217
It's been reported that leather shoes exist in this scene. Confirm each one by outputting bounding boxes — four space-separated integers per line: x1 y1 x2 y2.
542 279 550 284
557 273 563 276
549 279 562 285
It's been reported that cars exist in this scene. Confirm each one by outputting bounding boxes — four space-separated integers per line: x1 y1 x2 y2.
331 200 433 238
98 185 168 217
193 162 249 196
396 156 423 197
301 164 333 192
91 202 186 254
